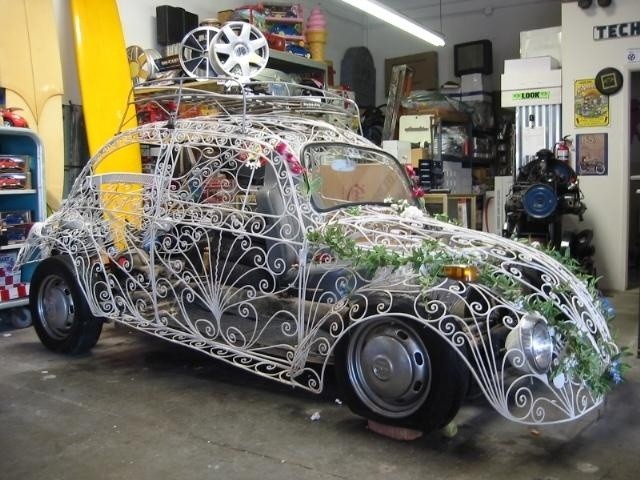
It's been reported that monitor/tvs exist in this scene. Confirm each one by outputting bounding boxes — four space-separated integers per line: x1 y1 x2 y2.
454 40 492 74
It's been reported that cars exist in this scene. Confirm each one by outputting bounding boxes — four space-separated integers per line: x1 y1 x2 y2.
11 76 633 432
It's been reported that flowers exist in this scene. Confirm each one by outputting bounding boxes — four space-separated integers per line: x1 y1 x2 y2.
276 142 307 173
406 166 424 197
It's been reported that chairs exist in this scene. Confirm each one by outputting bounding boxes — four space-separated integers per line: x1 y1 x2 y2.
256 151 373 303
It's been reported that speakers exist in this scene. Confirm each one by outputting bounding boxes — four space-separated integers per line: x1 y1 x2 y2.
184 12 198 38
156 6 184 45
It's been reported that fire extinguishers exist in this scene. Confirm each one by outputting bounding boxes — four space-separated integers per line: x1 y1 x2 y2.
553 135 572 164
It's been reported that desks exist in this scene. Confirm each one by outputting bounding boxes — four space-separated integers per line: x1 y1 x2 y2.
423 194 478 230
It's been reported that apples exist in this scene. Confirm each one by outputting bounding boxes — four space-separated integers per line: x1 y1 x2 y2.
270 37 285 50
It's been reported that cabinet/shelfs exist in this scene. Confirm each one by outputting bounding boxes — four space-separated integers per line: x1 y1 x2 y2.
407 108 516 231
0 126 45 327
231 48 328 96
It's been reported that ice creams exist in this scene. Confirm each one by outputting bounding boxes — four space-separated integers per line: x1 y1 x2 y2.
305 7 327 61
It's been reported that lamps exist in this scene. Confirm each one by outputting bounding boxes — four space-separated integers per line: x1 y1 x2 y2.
343 0 446 47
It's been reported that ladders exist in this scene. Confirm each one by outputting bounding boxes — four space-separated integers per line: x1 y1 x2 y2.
380 64 416 147
